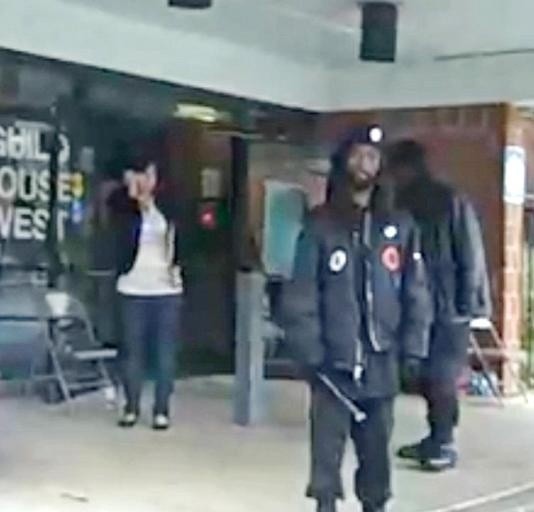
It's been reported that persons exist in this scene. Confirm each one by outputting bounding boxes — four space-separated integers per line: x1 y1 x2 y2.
384 139 492 471
282 122 435 512
106 145 187 429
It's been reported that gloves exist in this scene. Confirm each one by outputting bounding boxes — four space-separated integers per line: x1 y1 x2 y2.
400 358 423 394
316 356 353 383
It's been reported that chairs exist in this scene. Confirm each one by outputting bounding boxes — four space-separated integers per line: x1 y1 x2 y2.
26 291 117 410
466 317 530 401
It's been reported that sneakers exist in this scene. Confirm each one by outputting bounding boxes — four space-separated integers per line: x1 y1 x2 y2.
153 409 169 429
119 409 138 426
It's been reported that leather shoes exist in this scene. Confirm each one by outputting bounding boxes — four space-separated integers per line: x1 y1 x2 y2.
398 441 457 472
